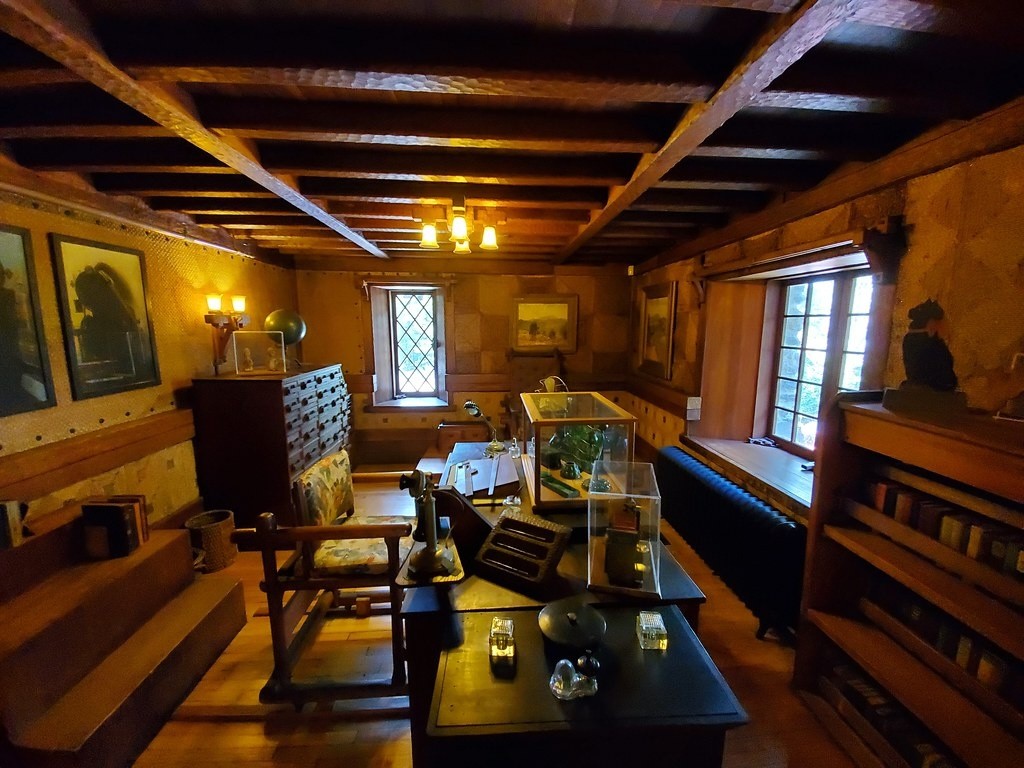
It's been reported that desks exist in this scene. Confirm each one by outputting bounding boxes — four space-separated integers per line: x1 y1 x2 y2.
426 604 750 768
400 441 706 768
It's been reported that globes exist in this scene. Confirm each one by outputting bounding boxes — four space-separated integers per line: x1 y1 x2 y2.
264 309 307 370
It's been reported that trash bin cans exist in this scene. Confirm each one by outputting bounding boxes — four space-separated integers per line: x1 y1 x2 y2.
184 510 238 574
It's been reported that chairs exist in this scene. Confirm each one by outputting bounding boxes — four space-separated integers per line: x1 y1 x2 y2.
289 447 415 613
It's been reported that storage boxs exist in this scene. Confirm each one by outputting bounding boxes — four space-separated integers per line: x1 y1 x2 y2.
587 460 663 602
518 393 637 514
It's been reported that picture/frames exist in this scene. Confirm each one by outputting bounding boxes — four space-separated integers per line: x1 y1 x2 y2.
638 281 677 381
508 293 579 356
0 222 56 419
47 231 162 401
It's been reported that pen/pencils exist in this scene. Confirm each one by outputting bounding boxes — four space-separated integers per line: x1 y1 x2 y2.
510 484 525 501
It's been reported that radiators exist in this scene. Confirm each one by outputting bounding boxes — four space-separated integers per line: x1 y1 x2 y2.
655 447 809 640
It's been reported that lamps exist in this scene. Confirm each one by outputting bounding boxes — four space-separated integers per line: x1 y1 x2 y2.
412 196 508 253
463 402 505 458
204 293 251 375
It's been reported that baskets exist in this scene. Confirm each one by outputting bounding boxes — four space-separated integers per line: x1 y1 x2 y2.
185 510 239 574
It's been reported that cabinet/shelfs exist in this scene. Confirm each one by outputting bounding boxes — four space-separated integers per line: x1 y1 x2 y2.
192 362 352 552
790 387 1024 768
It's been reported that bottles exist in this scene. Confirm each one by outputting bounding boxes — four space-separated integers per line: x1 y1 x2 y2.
509 438 520 458
527 437 535 458
